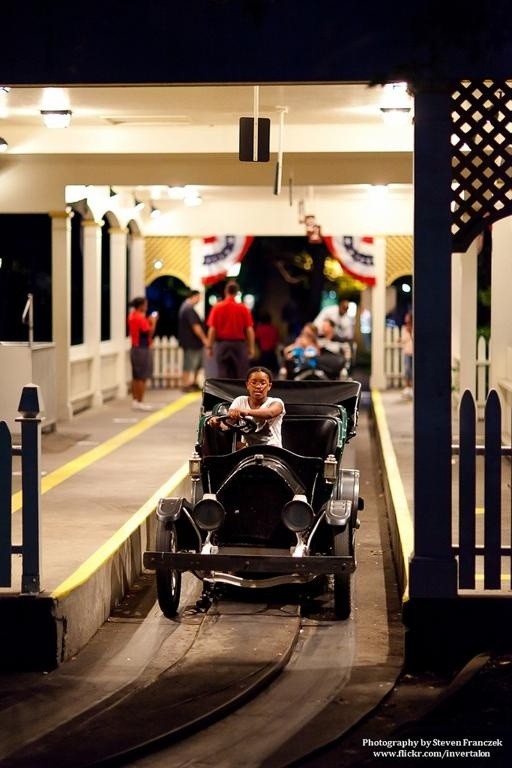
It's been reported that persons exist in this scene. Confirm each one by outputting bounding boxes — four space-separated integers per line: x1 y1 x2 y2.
206 283 254 378
285 320 356 377
207 366 285 453
127 297 159 408
313 301 354 337
396 314 414 399
179 290 210 393
358 302 371 355
254 311 283 373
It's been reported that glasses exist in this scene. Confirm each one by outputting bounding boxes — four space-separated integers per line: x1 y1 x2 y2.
248 381 269 387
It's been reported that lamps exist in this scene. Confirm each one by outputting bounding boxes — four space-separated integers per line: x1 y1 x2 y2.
109 185 161 217
380 107 411 124
41 110 72 129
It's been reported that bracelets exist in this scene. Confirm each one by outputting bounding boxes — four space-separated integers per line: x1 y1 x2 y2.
208 346 213 348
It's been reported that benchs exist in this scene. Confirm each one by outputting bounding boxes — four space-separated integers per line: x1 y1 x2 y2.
203 403 344 484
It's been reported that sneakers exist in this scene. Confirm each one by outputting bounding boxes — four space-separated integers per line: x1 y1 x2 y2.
131 400 152 411
